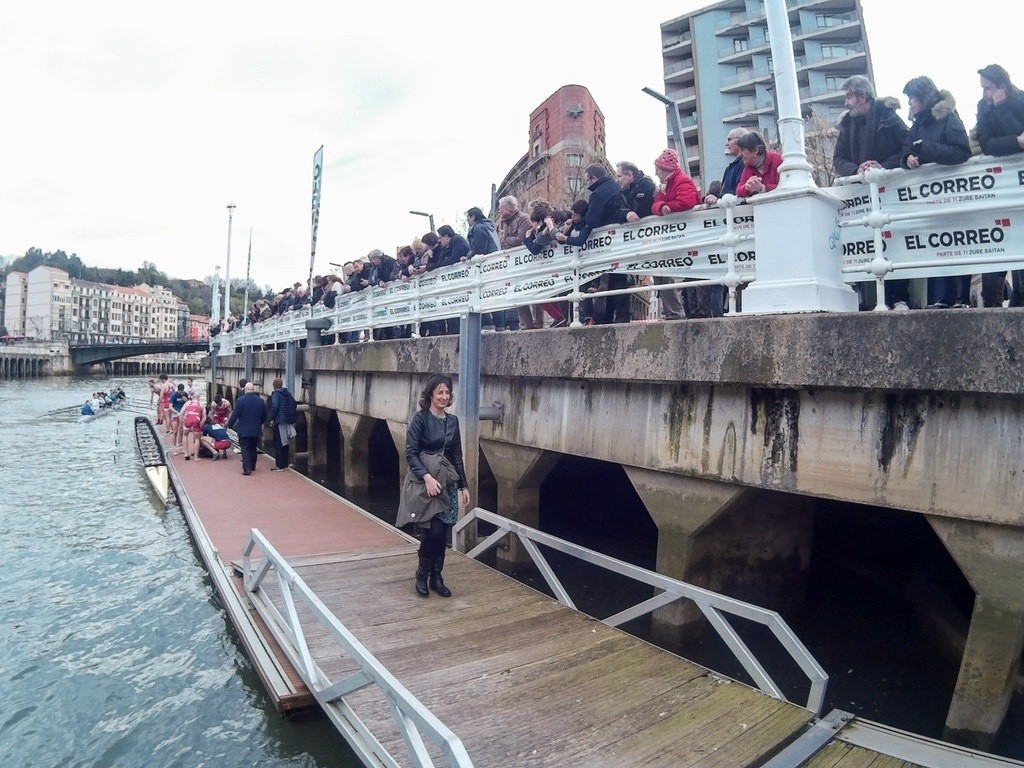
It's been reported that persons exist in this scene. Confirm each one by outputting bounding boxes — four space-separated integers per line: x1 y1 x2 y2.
148 374 232 461
468 127 783 330
269 378 297 472
82 387 125 415
832 64 1024 311
395 374 470 598
235 379 259 443
226 382 265 475
210 225 469 353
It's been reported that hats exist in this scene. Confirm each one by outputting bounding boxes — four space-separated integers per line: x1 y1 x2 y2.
653 149 681 172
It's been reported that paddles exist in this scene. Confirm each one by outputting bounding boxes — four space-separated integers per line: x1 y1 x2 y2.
128 397 158 405
128 400 157 407
123 403 158 411
33 402 87 419
105 405 150 416
227 434 265 454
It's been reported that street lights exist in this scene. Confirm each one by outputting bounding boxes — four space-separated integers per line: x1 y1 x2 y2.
409 211 437 234
640 85 693 176
225 201 236 319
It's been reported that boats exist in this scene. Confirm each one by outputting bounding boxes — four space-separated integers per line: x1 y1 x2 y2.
78 400 125 424
134 415 169 508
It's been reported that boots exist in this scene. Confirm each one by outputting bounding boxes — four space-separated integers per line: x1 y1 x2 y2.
429 553 451 597
415 545 432 596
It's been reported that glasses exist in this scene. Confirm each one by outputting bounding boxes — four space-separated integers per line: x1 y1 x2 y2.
727 137 741 142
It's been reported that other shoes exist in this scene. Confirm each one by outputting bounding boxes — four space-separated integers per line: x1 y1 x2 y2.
184 456 190 461
893 300 910 313
222 453 227 459
234 305 635 352
212 453 220 461
241 471 250 475
924 300 949 310
155 416 186 456
249 468 257 471
953 302 972 309
274 464 288 470
271 467 284 472
193 458 201 461
